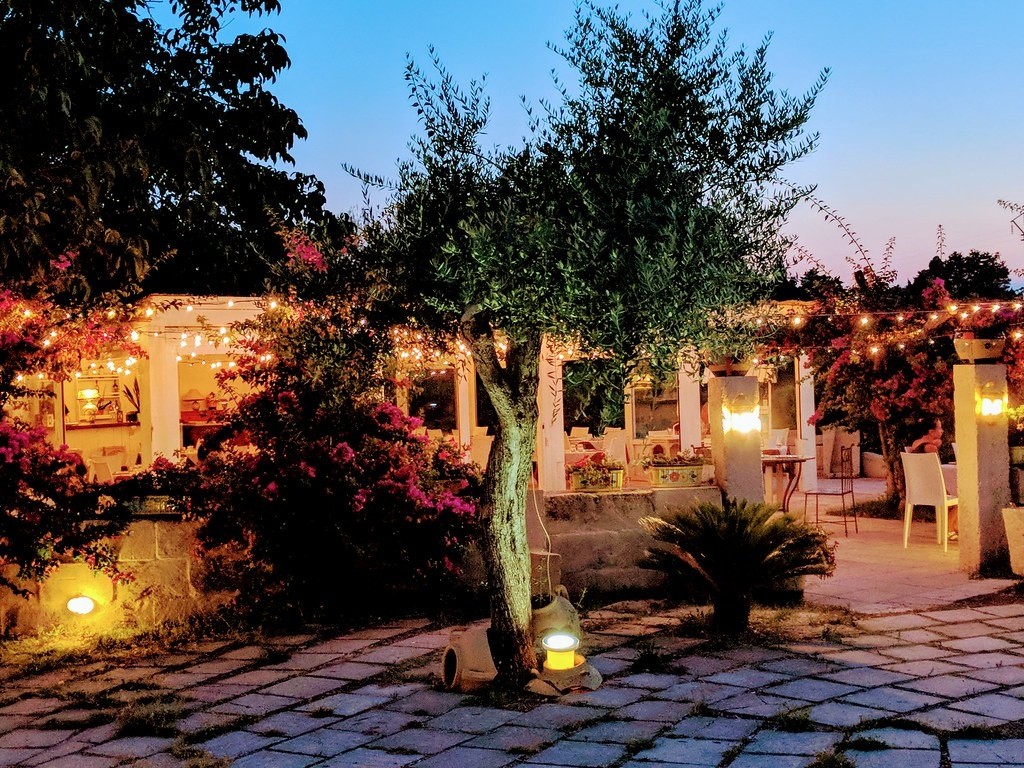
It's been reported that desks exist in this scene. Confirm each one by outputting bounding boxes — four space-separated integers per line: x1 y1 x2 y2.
179 444 200 467
178 417 239 443
940 460 958 497
563 448 607 466
758 454 815 514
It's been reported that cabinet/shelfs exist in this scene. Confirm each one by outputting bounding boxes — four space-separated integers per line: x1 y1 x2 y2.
76 357 123 422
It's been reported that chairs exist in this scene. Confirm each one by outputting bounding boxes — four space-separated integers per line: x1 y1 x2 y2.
899 450 958 557
803 443 859 539
1000 507 1024 578
411 424 497 469
762 449 800 493
564 424 701 468
64 444 143 485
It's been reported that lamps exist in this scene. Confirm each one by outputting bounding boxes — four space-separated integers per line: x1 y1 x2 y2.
76 387 102 424
719 389 763 438
972 378 1009 419
540 631 581 672
183 387 207 413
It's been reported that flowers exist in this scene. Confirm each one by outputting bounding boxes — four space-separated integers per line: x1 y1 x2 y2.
1008 405 1024 446
566 457 628 494
632 449 708 471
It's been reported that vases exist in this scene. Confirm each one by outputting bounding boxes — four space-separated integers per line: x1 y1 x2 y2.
1009 446 1024 466
646 461 705 488
570 471 624 492
